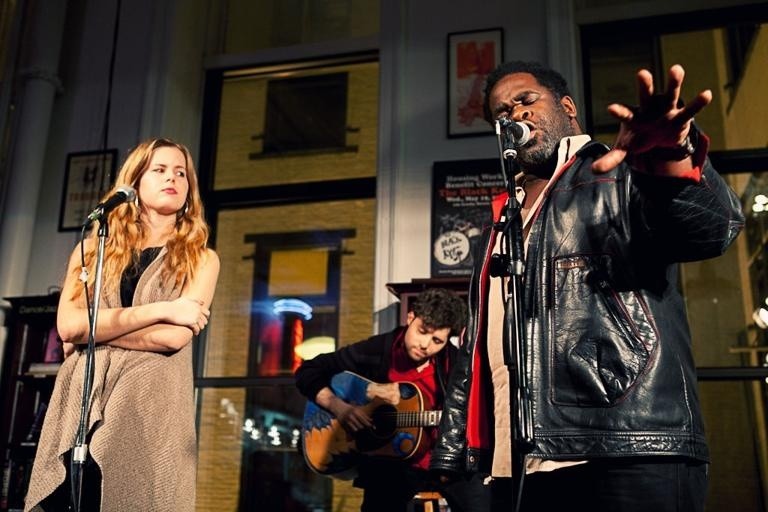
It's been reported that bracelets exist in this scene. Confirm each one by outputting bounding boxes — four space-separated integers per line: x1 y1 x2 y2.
643 137 694 164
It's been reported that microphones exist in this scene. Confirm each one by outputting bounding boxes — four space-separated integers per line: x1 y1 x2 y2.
83 186 135 230
498 116 530 148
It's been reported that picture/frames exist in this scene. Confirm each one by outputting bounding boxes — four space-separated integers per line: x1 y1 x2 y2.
58 149 118 233
446 27 504 140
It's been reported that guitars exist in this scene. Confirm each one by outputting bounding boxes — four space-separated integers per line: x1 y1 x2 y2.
303 371 443 480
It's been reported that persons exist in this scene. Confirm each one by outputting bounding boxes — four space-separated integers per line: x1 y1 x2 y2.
295 288 472 511
424 55 747 509
23 135 220 511
